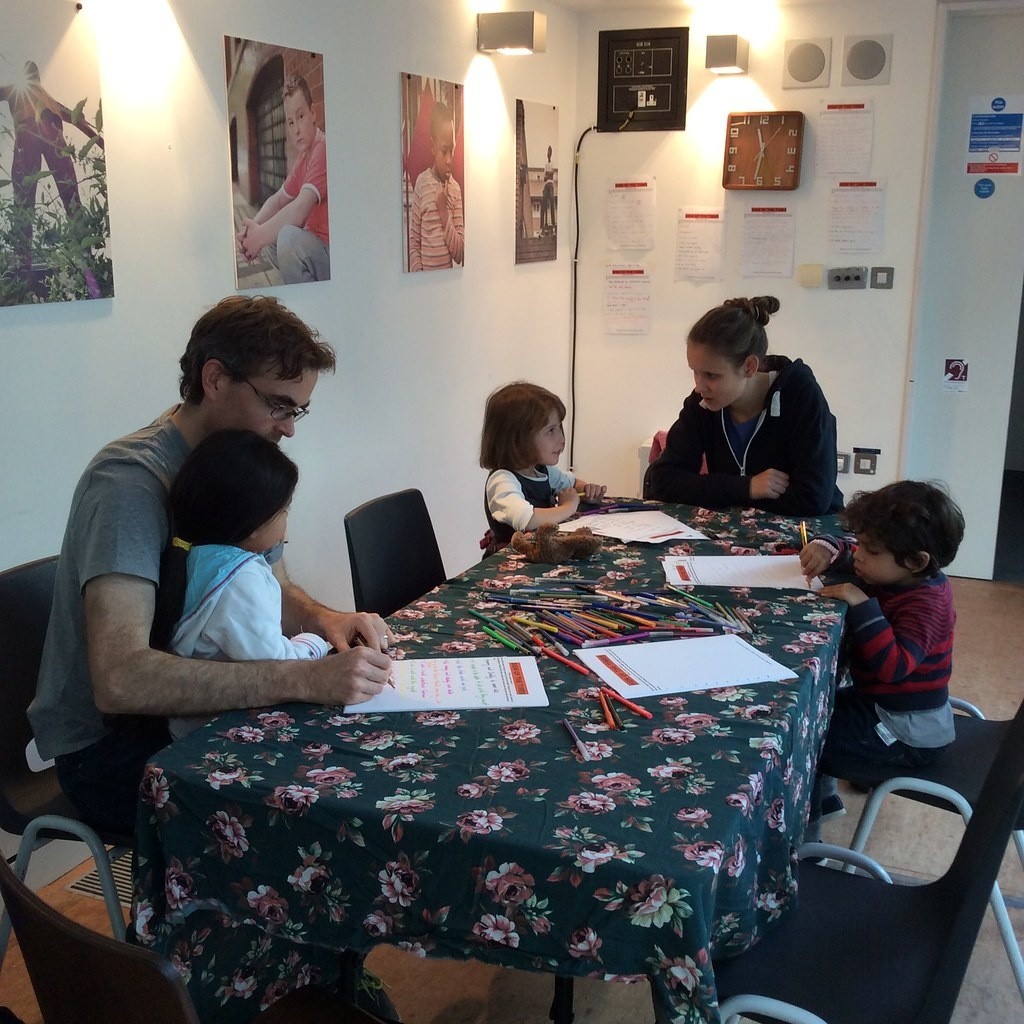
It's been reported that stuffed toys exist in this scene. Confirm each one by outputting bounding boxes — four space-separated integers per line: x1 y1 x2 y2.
511 520 603 564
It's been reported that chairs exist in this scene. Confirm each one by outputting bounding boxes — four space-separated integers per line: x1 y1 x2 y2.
0 841 199 1024
713 697 1024 1024
817 689 1024 999
344 488 447 622
0 554 158 972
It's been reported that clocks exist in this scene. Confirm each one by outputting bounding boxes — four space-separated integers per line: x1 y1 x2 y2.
722 111 806 191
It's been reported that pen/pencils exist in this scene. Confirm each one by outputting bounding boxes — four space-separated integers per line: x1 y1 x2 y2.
600 687 653 720
541 647 590 676
577 492 586 496
599 691 615 729
563 718 591 762
354 637 395 689
467 583 761 659
605 694 625 731
582 502 660 515
534 577 601 585
800 520 812 588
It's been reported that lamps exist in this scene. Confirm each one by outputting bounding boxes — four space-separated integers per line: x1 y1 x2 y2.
477 11 547 56
704 35 748 74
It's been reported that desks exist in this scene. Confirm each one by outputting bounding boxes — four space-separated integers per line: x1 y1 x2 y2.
125 496 853 1024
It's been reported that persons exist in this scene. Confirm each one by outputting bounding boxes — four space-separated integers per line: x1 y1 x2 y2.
27 295 397 846
409 101 464 272
238 72 331 284
643 295 844 517
149 428 329 743
480 379 607 560
0 60 105 283
538 146 556 238
799 480 966 867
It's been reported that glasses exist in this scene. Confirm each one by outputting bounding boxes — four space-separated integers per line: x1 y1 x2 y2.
244 374 309 422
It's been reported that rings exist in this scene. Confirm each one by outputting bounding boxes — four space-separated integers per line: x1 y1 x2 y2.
384 635 388 639
